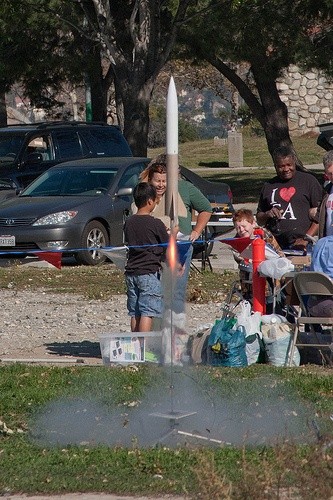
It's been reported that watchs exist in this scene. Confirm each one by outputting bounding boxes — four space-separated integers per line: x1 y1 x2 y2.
303 236 312 242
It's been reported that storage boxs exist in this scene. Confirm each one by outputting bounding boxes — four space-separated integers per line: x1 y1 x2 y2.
98 333 164 368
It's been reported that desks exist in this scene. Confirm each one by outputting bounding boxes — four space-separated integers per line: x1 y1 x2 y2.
211 202 235 234
192 221 234 271
281 246 310 255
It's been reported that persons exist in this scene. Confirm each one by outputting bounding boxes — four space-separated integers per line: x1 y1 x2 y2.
124 183 179 363
256 146 326 256
131 164 179 242
231 209 294 316
156 152 213 316
295 150 333 365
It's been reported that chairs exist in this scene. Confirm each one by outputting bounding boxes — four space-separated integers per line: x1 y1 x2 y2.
121 174 138 197
219 229 333 367
32 141 49 161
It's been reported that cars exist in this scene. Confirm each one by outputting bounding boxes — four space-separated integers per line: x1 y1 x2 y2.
0 157 236 266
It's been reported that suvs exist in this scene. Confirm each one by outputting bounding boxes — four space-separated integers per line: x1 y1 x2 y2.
0 121 134 205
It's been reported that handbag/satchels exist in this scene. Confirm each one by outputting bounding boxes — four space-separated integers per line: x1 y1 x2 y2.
188 300 300 368
